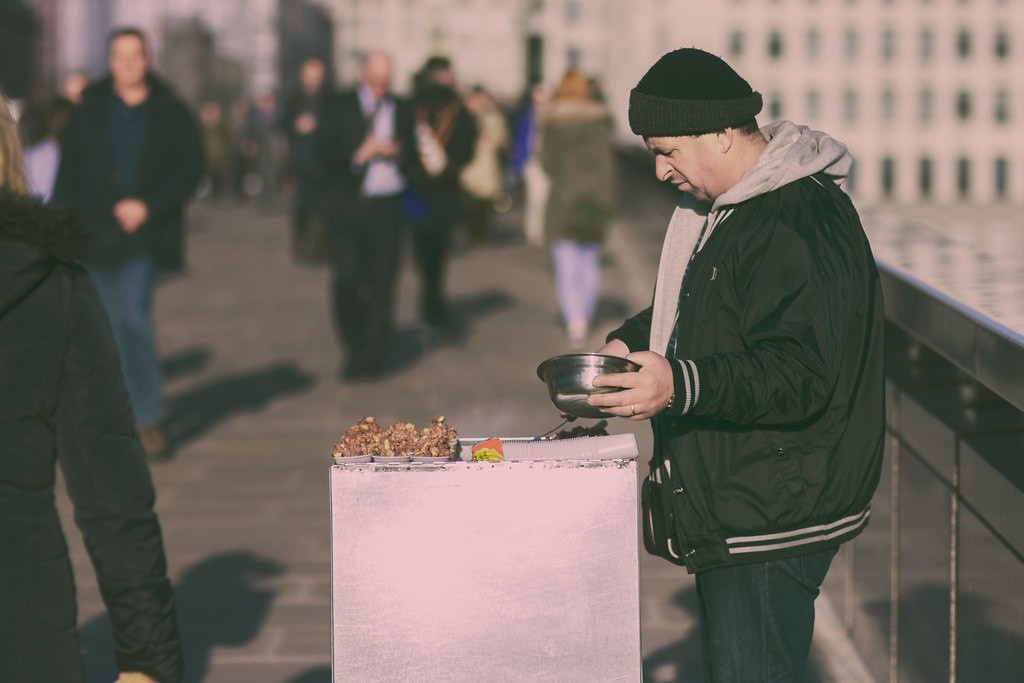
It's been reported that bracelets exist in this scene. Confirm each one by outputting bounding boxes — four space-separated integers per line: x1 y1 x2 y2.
671 396 681 416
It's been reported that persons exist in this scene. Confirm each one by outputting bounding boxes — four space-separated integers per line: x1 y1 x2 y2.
561 47 885 683
0 94 184 682
60 31 203 461
206 56 616 379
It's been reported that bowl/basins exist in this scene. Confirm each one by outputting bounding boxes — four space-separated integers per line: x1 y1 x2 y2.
537 352 641 419
412 455 451 463
371 454 411 463
334 453 372 465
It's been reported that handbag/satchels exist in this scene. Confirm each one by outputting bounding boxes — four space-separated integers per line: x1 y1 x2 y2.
524 172 552 246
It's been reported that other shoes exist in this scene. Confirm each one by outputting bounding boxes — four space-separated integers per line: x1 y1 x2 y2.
566 315 592 339
139 425 170 456
421 297 447 329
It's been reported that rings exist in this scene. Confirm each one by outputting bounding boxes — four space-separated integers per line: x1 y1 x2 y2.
631 404 635 416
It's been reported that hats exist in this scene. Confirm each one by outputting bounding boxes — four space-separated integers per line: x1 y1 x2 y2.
628 48 763 136
